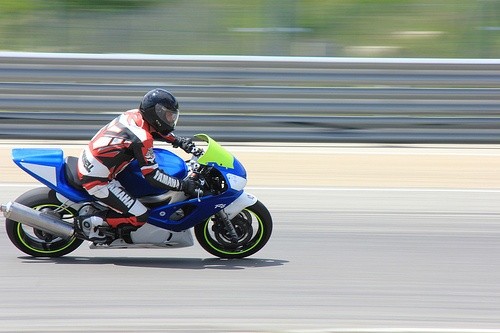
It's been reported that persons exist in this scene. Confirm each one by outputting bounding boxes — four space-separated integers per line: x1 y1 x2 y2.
74 88 204 241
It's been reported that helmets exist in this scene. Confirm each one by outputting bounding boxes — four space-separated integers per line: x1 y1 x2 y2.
139 88 180 136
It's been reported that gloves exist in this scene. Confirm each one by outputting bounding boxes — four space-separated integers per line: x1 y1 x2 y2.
179 137 194 152
181 180 203 197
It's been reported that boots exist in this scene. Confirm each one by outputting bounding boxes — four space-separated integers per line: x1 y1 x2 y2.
88 216 137 241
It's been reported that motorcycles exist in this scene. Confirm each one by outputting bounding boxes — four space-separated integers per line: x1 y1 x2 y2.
0 132 274 260
